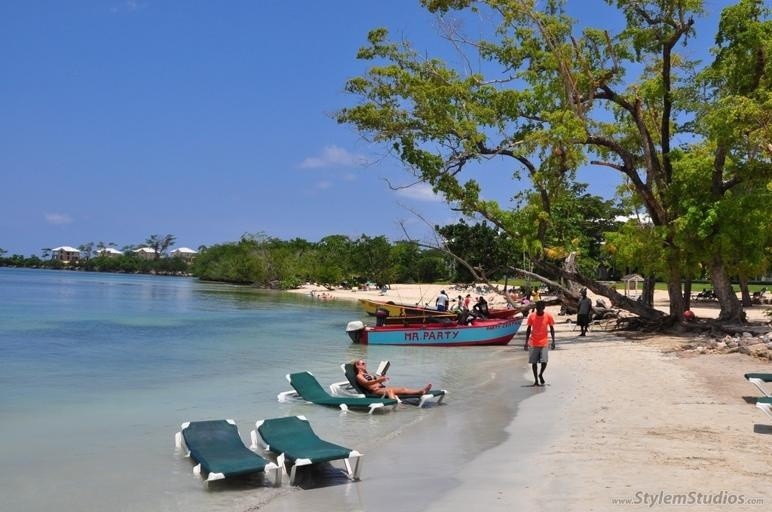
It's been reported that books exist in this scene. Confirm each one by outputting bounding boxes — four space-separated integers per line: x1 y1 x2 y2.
376 361 390 376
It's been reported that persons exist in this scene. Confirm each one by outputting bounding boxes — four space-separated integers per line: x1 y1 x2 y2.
506 285 541 314
312 290 334 299
576 288 591 336
435 290 489 316
354 359 431 396
524 299 556 385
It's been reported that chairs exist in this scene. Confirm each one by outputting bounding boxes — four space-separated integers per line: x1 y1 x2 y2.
174 358 448 487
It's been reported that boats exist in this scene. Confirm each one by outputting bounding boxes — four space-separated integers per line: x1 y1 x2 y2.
344 298 525 347
